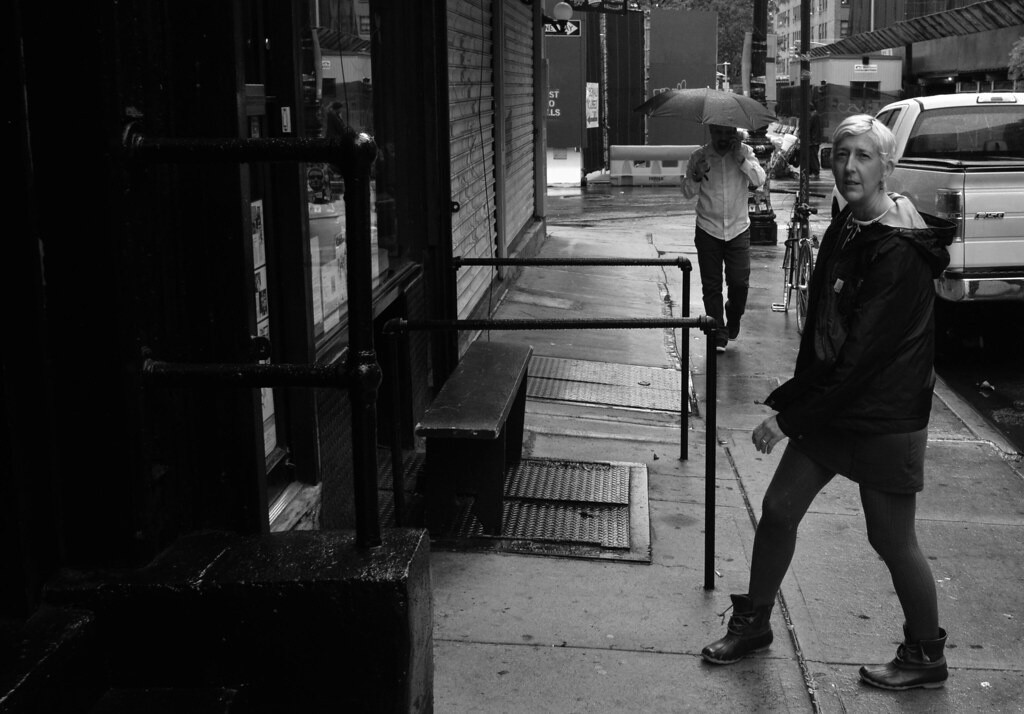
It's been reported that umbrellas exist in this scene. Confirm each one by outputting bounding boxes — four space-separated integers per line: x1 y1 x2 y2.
633 85 780 173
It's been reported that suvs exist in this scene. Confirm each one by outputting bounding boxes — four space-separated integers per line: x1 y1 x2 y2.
830 89 1024 303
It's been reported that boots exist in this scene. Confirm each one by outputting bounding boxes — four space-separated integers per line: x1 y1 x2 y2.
859 622 948 690
702 591 776 663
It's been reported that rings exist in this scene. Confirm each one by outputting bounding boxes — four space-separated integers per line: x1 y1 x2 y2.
762 439 768 443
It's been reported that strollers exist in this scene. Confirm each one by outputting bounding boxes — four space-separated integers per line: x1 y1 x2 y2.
765 132 800 180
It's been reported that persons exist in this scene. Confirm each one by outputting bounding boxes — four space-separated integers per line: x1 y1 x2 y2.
679 123 768 350
809 102 823 180
703 114 957 690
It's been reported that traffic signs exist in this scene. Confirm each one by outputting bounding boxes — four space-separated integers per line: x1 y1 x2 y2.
545 19 582 37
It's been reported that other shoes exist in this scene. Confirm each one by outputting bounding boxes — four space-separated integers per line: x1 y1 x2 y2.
716 336 726 353
726 316 740 341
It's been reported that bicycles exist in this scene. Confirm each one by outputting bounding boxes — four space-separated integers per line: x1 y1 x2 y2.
769 188 827 336
